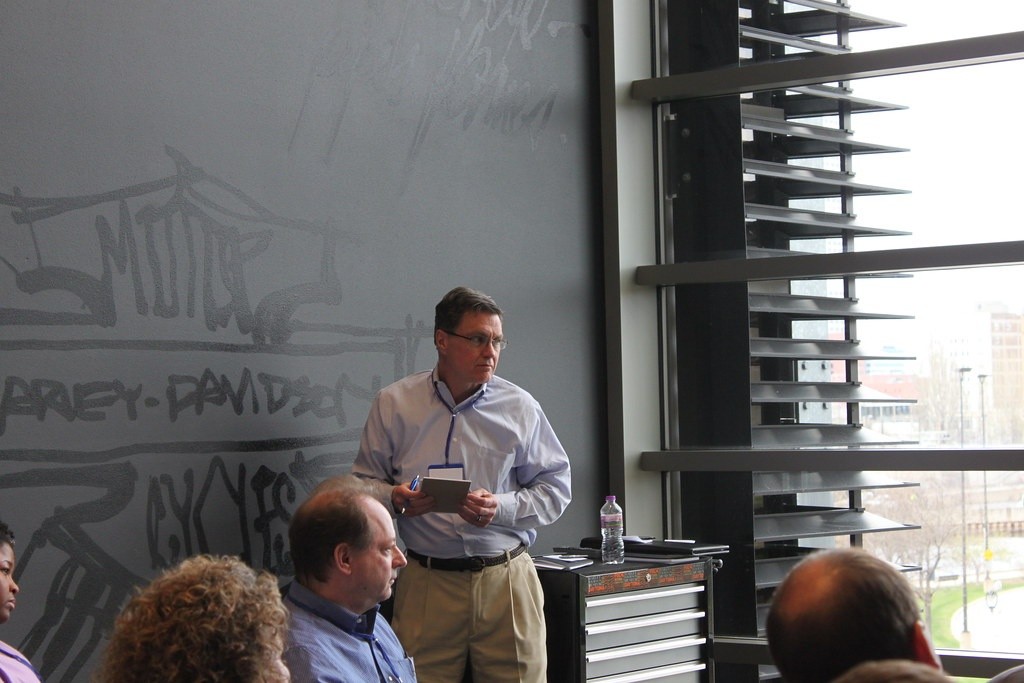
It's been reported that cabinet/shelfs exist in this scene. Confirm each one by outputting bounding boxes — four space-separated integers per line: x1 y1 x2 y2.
538 556 724 683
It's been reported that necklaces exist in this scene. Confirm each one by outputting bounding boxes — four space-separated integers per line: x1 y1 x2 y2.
0 648 44 682
286 591 403 682
92 553 291 683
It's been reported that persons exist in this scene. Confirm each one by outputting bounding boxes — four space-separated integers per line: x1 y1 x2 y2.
766 547 1023 682
0 519 43 683
348 287 570 682
276 475 418 683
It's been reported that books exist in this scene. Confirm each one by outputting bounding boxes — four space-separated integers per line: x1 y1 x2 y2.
530 552 595 571
579 536 730 565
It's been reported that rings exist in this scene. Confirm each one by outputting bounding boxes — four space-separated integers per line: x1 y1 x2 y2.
476 516 481 522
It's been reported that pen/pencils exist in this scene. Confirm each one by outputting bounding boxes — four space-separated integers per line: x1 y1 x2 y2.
401 474 421 514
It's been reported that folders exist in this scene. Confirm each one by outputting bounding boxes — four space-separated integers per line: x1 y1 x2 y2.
580 535 730 556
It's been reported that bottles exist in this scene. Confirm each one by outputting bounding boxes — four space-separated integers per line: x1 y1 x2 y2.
600 495 625 564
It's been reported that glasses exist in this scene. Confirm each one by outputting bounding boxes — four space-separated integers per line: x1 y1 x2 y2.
447 330 508 352
